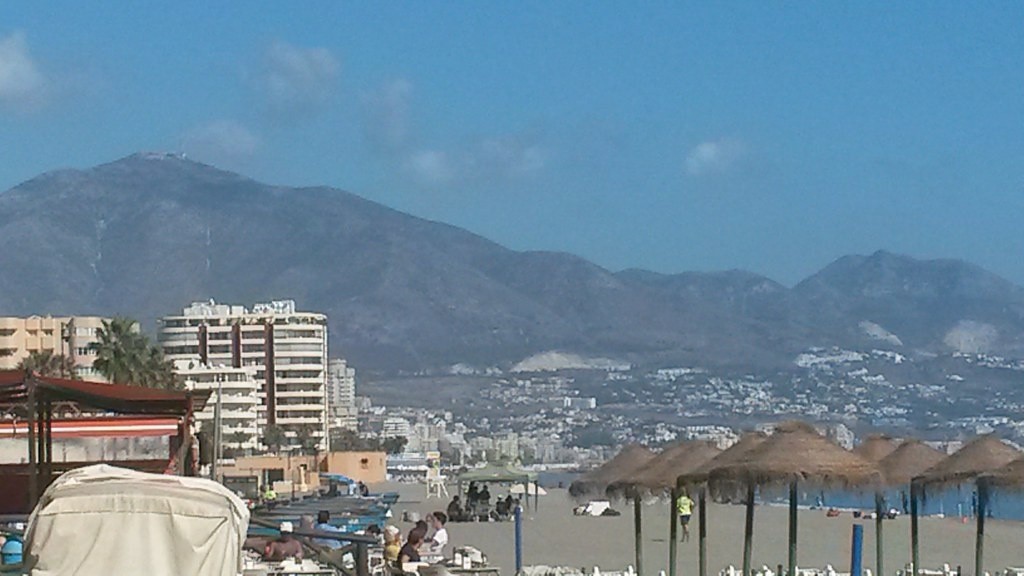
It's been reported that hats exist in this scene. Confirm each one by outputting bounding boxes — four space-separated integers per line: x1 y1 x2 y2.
280 522 292 532
498 494 503 498
384 525 399 542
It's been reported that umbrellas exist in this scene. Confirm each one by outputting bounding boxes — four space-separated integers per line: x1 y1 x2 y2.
567 416 1024 576
509 480 547 498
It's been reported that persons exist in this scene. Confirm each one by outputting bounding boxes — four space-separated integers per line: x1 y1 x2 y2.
675 490 696 544
246 480 522 576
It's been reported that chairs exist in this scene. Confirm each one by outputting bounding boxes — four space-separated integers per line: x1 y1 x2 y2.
386 560 421 576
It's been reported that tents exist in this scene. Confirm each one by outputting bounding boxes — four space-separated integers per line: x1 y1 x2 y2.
457 462 539 512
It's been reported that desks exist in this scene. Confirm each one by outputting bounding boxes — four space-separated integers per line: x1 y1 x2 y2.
243 542 501 576
